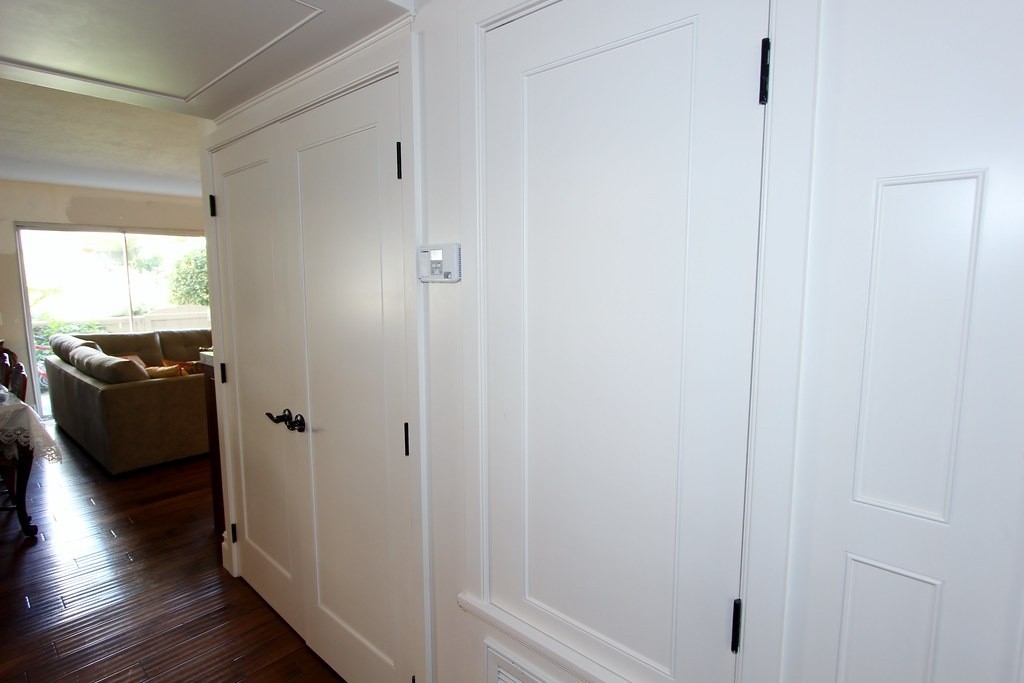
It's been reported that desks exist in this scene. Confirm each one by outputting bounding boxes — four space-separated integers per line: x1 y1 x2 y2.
0 383 62 537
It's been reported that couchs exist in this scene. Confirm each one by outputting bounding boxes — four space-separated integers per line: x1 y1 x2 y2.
44 328 216 474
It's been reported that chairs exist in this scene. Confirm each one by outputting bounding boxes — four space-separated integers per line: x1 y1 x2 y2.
0 339 27 512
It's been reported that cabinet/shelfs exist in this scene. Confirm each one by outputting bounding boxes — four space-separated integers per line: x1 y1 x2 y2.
200 1 1024 683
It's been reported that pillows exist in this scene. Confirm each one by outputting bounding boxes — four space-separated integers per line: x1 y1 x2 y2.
118 355 200 379
